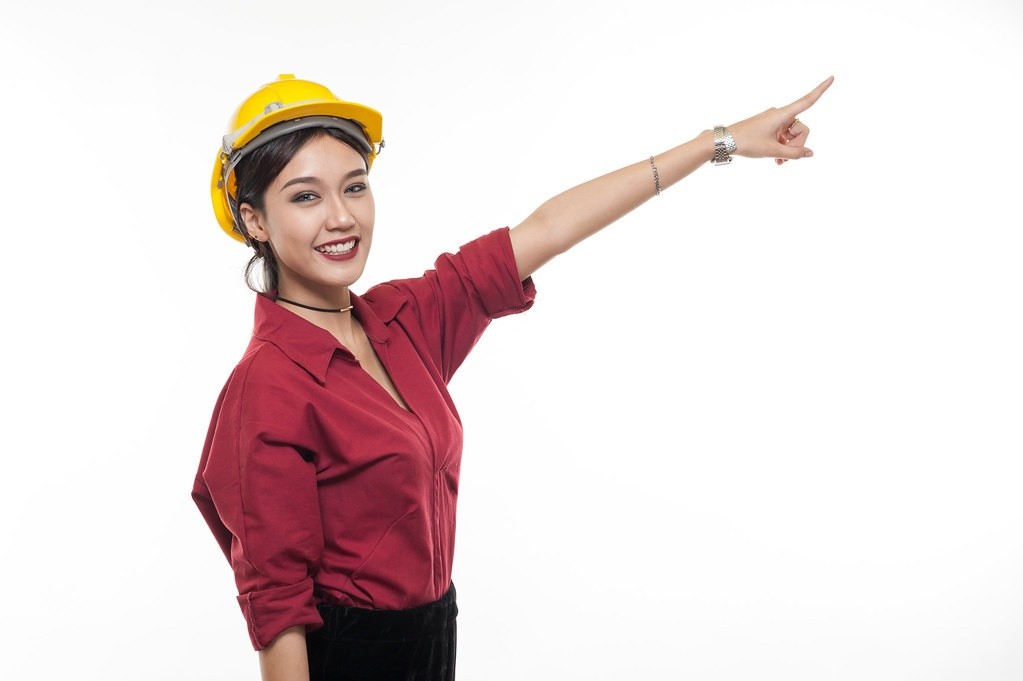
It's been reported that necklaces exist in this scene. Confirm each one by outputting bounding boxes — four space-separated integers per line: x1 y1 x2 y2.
275 293 354 312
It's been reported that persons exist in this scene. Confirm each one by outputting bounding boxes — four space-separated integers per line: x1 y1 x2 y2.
192 73 838 681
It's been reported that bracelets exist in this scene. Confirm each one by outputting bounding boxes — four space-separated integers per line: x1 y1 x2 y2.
648 154 661 196
711 125 737 166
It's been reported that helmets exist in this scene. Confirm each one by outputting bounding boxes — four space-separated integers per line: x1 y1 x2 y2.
211 74 384 248
